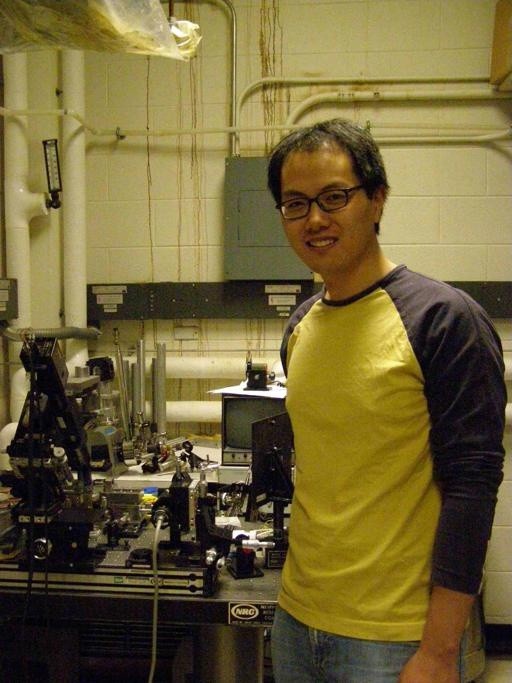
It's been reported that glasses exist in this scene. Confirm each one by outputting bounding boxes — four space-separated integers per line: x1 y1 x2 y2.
275 184 364 219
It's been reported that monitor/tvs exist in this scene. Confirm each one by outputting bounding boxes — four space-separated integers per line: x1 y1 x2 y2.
250 411 294 493
221 393 296 468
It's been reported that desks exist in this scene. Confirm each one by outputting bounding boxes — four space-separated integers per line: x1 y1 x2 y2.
0 514 291 683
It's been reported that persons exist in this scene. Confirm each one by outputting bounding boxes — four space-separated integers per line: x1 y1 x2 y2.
267 116 507 682
